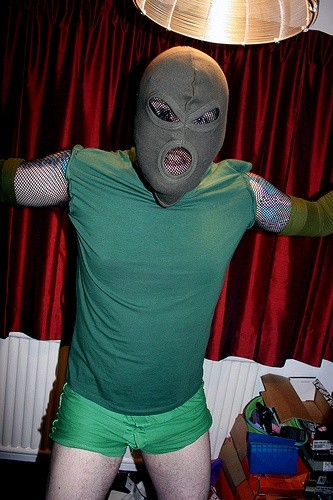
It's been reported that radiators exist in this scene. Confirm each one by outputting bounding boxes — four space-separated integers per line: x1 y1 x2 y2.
0 331 261 472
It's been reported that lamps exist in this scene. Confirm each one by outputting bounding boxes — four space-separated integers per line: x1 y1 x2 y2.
133 0 320 46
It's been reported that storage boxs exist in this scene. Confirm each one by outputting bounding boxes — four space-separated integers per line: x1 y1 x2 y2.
214 373 333 500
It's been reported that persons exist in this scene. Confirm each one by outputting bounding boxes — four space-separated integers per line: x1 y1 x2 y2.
0 46 333 500
